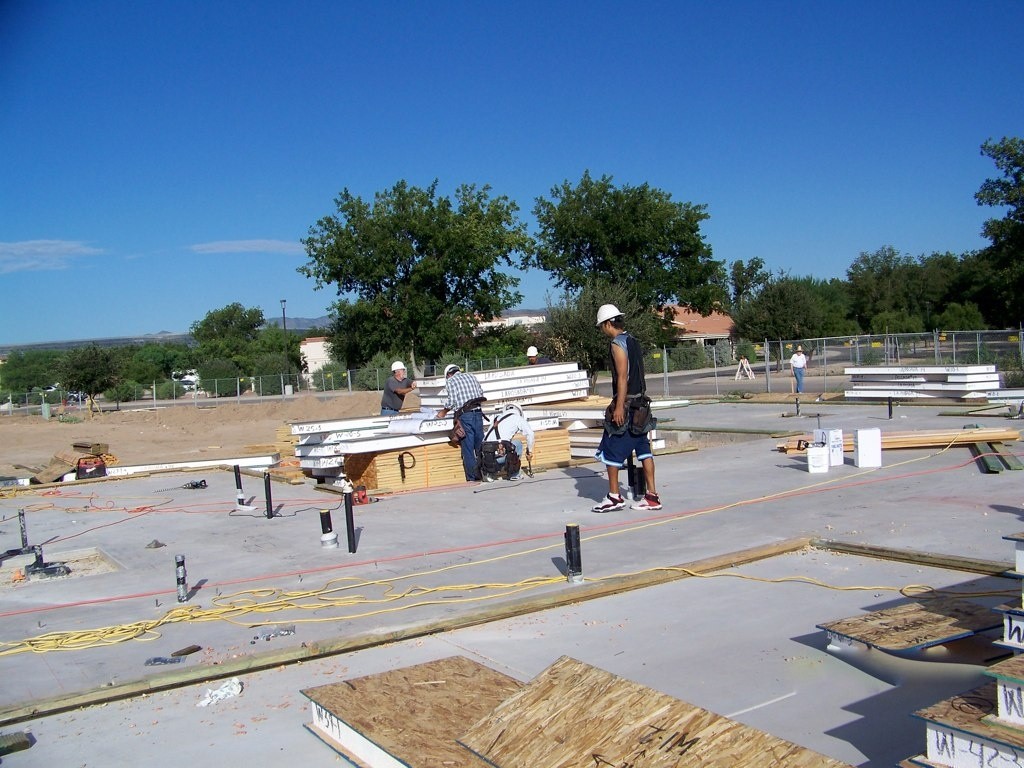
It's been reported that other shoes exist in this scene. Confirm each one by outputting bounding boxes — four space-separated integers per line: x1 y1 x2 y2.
510 474 524 480
480 470 494 482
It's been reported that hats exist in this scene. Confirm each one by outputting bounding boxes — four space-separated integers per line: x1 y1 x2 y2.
795 346 804 352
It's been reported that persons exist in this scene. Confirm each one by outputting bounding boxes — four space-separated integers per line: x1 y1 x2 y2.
379 360 417 417
524 345 554 367
435 363 488 485
479 400 535 483
591 303 663 515
788 344 809 394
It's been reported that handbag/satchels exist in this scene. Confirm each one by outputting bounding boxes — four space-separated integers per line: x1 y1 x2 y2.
479 441 501 473
502 440 521 476
603 401 630 435
448 421 466 445
631 397 658 434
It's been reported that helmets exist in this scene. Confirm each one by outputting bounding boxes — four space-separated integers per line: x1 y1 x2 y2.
391 361 405 371
596 304 625 327
444 364 461 379
526 346 538 357
503 403 523 416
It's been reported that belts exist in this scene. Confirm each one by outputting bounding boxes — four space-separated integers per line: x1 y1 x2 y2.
468 409 482 413
382 407 398 412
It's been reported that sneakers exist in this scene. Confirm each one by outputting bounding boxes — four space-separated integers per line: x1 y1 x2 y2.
592 492 627 512
631 490 662 512
